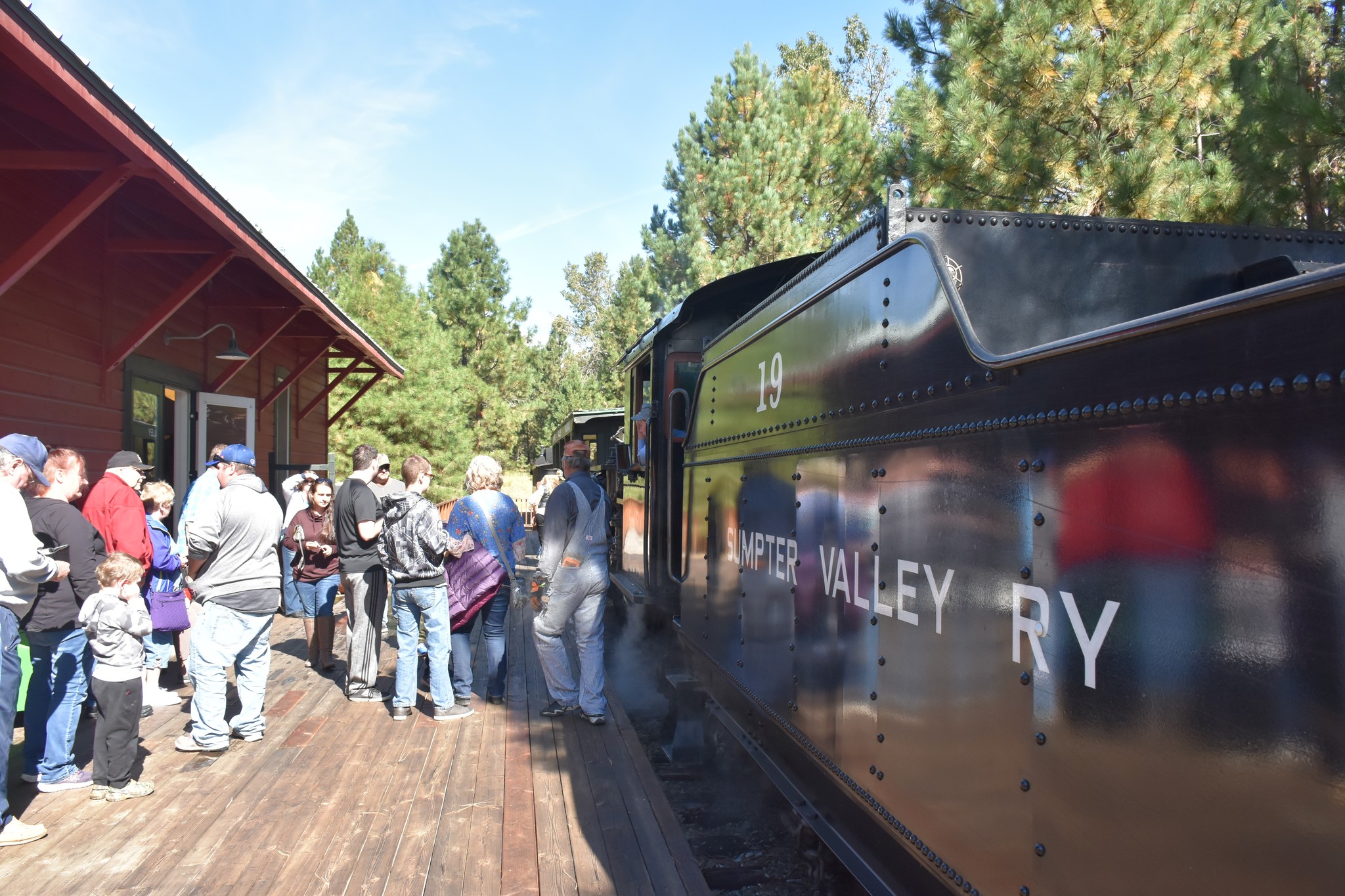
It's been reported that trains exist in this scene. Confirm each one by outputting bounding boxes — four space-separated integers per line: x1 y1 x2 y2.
531 182 1345 896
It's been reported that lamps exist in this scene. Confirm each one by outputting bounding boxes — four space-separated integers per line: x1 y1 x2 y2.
161 323 250 360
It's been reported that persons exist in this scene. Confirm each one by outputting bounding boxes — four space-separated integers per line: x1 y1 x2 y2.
529 439 614 726
366 454 406 633
529 468 566 559
446 456 524 705
81 450 153 718
139 479 188 708
635 403 687 466
21 444 107 791
283 470 319 618
0 433 49 846
333 444 392 701
282 477 339 669
377 454 475 720
77 551 154 804
174 444 284 752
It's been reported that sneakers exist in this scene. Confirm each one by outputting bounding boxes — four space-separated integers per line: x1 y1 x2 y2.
540 701 606 725
20 769 92 793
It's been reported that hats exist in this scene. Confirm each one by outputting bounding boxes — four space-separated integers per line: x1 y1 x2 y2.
205 444 256 467
563 439 591 459
107 450 154 471
544 468 565 481
630 403 651 421
377 452 391 466
0 433 51 487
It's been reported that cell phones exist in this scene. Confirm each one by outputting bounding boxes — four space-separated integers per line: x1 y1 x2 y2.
562 556 580 567
314 541 325 551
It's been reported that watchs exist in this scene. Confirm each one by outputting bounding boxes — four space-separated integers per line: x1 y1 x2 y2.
533 578 546 586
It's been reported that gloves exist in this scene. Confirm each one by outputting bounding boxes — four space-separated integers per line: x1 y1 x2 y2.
529 578 546 613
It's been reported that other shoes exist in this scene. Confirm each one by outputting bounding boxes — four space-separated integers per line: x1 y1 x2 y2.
418 642 428 656
453 693 505 706
85 703 153 717
381 624 388 632
421 675 430 687
448 666 454 679
90 779 155 802
392 707 412 720
174 730 263 752
433 704 474 720
291 611 305 619
0 816 47 846
177 675 190 684
349 687 392 702
148 686 182 706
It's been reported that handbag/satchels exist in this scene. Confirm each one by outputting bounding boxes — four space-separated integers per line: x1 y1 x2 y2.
293 525 305 571
510 576 528 610
150 589 191 631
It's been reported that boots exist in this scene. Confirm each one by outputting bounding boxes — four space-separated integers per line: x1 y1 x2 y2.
304 615 336 669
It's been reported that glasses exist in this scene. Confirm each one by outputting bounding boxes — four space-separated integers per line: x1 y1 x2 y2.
22 462 35 484
212 453 229 466
425 472 435 480
315 477 333 485
379 466 390 470
129 468 145 474
